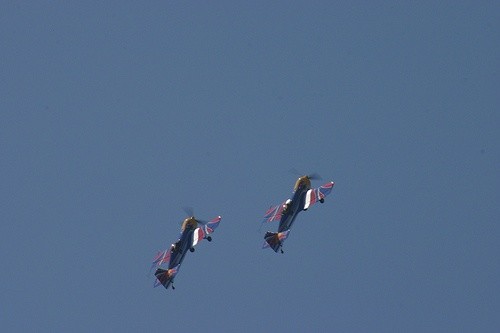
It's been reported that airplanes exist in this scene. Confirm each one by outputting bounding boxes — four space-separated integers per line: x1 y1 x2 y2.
258 175 334 256
148 216 224 290
